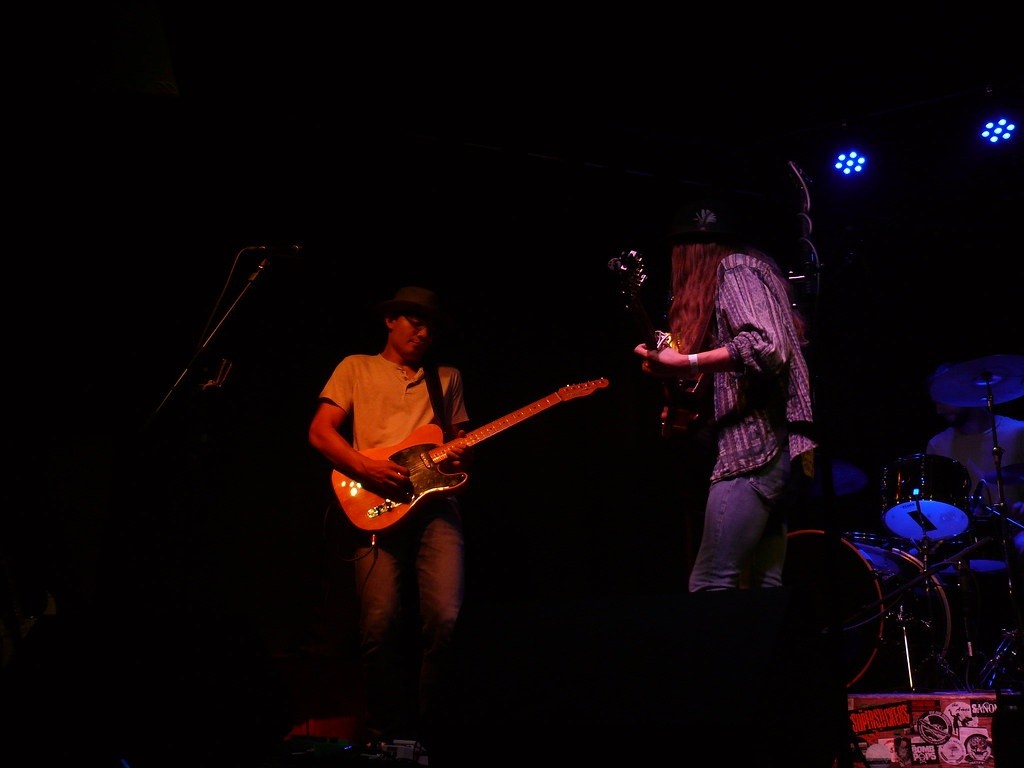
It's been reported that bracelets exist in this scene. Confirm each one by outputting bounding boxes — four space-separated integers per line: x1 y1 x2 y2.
689 353 700 376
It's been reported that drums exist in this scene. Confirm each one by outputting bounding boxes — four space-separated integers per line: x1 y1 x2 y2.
884 450 974 543
785 526 951 692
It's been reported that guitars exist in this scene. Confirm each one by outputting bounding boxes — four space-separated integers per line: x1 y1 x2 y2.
610 246 699 440
331 374 609 532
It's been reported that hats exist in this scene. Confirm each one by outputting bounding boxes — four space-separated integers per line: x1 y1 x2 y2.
381 286 442 321
669 202 739 244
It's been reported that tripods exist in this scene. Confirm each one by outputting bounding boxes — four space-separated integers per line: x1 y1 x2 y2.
974 387 1024 694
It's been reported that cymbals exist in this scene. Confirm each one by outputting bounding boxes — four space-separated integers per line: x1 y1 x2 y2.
930 353 1024 408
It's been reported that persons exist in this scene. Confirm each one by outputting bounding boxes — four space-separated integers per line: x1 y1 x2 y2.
634 198 818 598
308 287 477 726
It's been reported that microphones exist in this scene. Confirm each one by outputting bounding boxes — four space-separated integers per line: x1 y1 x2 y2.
262 244 303 256
